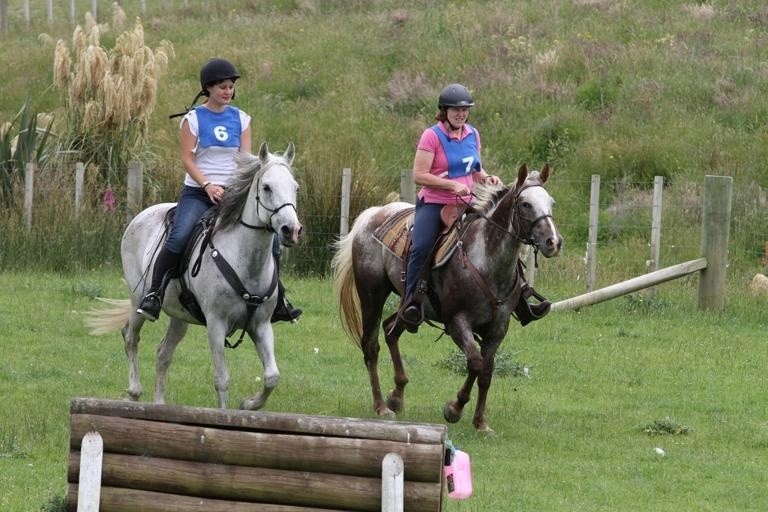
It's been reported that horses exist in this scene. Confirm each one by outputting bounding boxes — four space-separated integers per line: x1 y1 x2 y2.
80 141 303 409
328 161 564 433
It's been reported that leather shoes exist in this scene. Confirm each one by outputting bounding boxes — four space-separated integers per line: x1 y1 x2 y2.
271 300 301 323
516 298 551 326
137 287 166 321
403 296 420 333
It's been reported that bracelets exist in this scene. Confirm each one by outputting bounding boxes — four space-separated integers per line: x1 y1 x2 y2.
201 181 212 190
485 175 491 183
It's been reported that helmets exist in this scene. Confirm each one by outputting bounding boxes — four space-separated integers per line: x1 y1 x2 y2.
439 83 475 109
200 58 242 95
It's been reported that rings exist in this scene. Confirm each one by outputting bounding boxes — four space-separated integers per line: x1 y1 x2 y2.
216 192 221 196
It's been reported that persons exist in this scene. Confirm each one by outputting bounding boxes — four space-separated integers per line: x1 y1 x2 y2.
136 58 303 324
399 84 552 333
